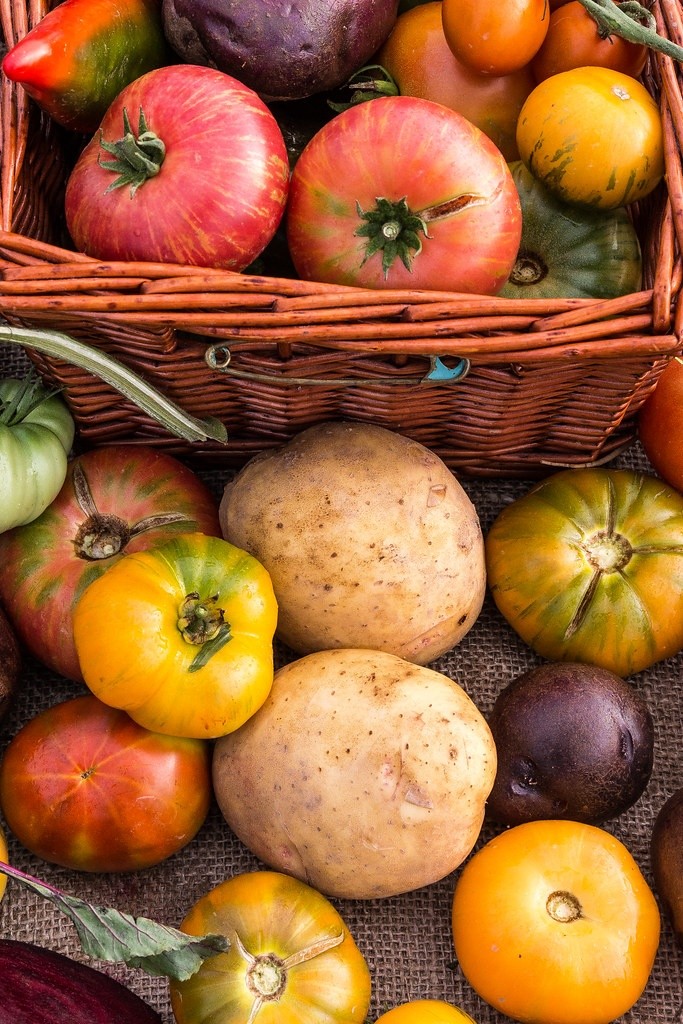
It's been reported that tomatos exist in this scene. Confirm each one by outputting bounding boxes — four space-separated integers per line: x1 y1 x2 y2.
63 0 667 302
0 351 683 1024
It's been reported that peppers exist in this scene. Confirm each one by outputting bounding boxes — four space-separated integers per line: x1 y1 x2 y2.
1 0 166 138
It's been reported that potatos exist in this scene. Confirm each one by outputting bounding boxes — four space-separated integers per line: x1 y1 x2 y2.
487 662 653 826
218 421 486 666
171 0 402 102
212 649 496 901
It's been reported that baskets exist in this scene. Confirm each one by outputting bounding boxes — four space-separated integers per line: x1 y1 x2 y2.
0 0 683 476
